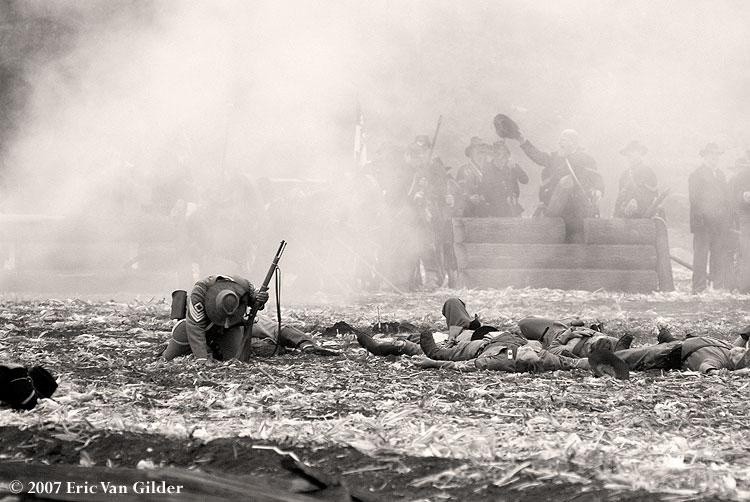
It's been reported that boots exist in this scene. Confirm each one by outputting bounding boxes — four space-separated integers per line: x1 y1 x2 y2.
419 330 444 360
357 334 403 357
300 342 340 356
367 266 464 295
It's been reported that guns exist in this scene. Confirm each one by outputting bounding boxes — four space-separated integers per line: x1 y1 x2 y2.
236 241 287 359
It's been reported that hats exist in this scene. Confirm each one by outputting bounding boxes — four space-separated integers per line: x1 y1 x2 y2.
588 350 629 381
733 349 750 371
204 282 248 328
406 113 647 158
699 143 725 157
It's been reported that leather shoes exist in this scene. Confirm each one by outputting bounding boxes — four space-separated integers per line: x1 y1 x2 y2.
615 334 633 351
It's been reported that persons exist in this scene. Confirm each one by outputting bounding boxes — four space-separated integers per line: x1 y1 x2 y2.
351 128 750 294
252 314 340 357
162 273 269 364
357 296 750 377
